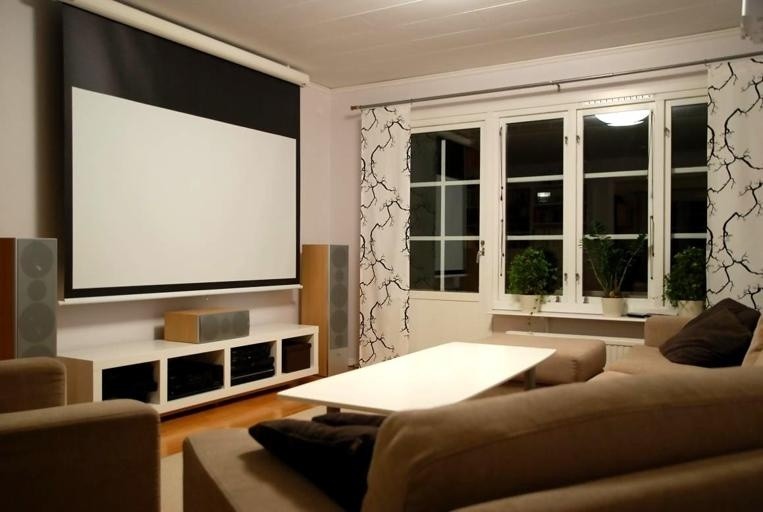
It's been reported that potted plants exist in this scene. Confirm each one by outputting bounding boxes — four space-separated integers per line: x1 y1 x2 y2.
506 245 558 333
578 219 647 317
662 247 707 317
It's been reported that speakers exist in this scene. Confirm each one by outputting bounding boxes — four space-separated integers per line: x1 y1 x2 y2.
301 244 350 378
0 238 58 360
164 306 250 344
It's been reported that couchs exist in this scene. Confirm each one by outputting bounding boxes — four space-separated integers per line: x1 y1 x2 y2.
180 310 763 512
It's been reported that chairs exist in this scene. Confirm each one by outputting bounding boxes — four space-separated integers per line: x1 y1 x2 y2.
0 357 162 512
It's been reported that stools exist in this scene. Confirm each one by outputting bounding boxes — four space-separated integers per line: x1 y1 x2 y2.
478 333 605 385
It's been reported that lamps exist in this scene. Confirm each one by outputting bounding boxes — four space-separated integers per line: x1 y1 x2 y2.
595 110 651 127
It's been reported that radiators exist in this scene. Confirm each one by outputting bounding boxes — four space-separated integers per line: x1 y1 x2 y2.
505 331 644 373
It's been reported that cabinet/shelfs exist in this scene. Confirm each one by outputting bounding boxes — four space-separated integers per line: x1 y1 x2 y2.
56 322 320 418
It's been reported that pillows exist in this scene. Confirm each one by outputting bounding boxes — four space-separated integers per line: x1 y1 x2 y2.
659 298 762 369
249 412 389 512
